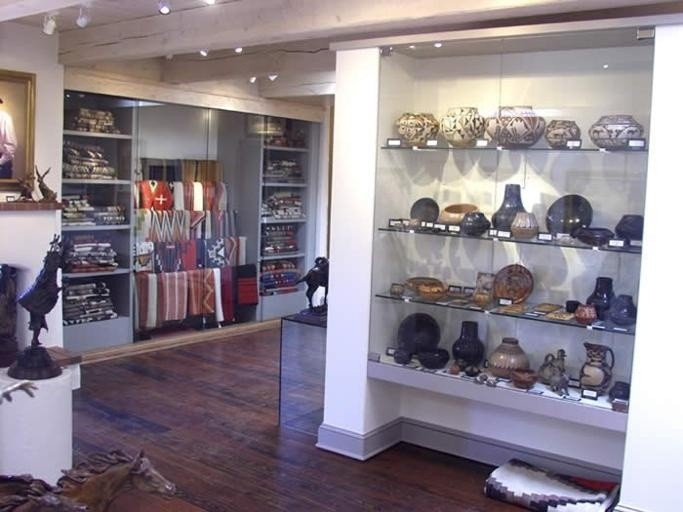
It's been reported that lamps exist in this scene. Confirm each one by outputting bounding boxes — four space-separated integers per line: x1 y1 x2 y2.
41 0 278 84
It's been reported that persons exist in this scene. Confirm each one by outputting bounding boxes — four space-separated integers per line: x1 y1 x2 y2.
0 98 17 178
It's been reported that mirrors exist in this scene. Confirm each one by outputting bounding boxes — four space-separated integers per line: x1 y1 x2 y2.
135 100 244 211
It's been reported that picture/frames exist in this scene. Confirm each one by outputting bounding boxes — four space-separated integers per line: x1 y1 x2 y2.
0 69 36 192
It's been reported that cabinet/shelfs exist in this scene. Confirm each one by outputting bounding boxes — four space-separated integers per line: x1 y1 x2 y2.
238 138 308 321
278 307 326 436
366 147 648 434
63 107 135 352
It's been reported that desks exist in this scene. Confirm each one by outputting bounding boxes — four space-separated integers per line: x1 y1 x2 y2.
0 346 81 490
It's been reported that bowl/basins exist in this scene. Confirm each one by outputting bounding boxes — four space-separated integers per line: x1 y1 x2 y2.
418 348 449 368
511 368 536 386
395 352 411 363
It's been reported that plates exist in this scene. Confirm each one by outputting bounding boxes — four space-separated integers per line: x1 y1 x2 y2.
410 198 439 230
398 313 440 351
495 265 533 304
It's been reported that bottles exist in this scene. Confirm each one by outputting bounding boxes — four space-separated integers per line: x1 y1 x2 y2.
546 194 592 236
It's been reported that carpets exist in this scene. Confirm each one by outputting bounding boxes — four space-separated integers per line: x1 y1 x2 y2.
11 489 207 512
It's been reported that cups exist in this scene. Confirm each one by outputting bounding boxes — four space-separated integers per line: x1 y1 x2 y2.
567 301 578 312
